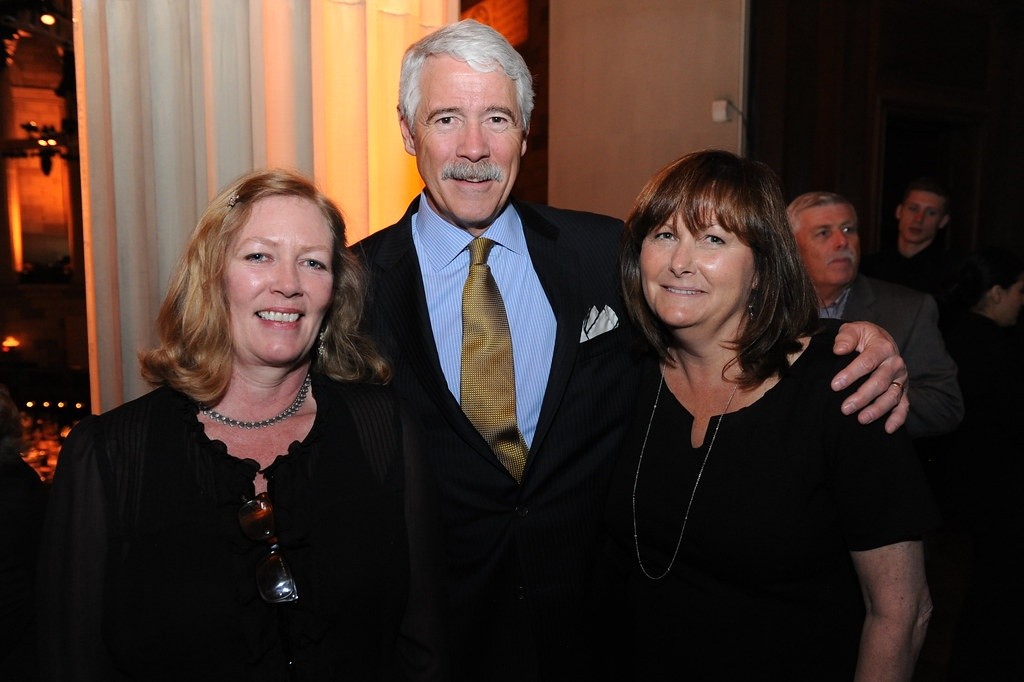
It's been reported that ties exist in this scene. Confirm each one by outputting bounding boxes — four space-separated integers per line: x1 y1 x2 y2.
459 237 529 485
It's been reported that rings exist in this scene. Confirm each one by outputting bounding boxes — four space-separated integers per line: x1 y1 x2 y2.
891 382 904 392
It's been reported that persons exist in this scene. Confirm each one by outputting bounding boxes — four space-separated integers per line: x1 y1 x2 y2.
575 149 936 681
314 19 918 682
873 180 954 297
784 190 964 444
937 244 1024 682
41 172 446 681
0 392 48 682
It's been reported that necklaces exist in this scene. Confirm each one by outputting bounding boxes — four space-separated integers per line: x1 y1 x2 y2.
200 369 312 426
631 345 737 578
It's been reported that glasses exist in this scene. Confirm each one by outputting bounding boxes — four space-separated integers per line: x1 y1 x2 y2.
238 493 300 604
